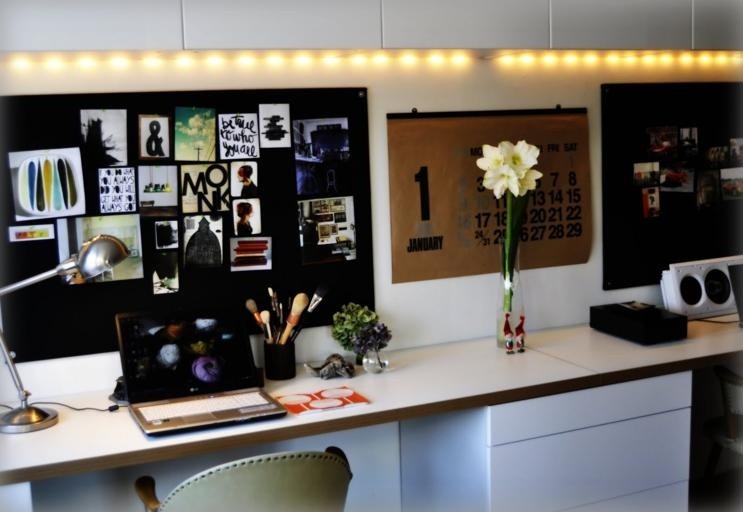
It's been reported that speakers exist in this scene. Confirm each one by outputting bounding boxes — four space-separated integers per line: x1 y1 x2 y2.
660 254 743 321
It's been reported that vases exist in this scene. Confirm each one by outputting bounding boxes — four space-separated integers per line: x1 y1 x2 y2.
495 239 527 352
362 349 387 373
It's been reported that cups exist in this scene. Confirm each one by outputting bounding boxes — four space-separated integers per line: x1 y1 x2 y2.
264 340 296 380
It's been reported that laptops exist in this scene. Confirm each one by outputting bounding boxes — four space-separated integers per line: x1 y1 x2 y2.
114 308 288 436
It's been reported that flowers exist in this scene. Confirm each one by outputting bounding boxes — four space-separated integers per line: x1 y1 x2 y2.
353 320 391 352
476 139 543 311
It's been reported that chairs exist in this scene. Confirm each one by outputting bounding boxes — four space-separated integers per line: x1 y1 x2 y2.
133 447 354 511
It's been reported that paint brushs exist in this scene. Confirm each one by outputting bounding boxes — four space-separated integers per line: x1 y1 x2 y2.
290 288 326 343
245 298 267 337
267 287 285 326
278 292 310 345
259 308 274 340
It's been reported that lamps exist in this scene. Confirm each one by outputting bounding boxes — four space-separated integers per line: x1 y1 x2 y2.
0 232 132 432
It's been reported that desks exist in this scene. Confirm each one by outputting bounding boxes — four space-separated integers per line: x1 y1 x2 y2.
0 312 742 510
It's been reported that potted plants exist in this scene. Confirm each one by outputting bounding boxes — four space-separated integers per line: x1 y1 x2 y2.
330 302 376 365
